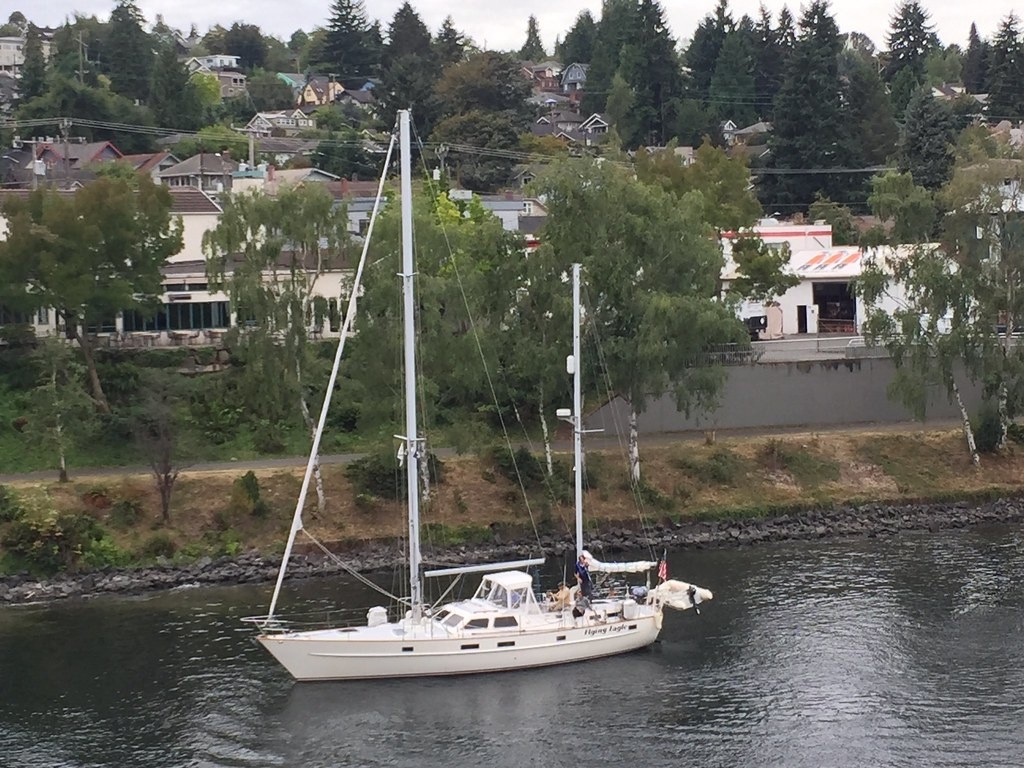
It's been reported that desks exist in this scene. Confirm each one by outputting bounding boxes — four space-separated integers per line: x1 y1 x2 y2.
97 336 107 348
177 333 187 345
211 332 222 343
139 335 152 347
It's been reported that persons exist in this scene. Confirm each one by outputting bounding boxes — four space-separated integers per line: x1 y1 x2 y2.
572 591 590 618
546 582 570 612
574 555 592 605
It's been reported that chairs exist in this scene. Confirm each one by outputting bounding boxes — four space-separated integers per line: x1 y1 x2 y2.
87 328 222 349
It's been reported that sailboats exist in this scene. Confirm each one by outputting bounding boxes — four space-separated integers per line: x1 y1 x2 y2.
241 109 711 682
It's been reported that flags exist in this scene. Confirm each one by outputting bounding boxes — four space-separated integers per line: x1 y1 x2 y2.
659 549 667 581
